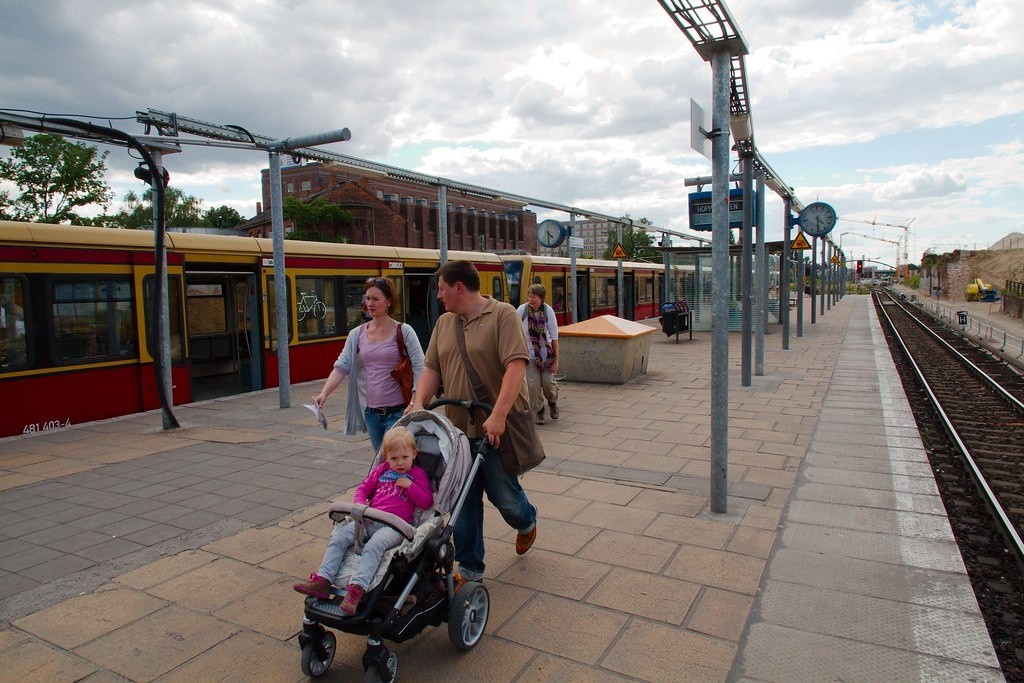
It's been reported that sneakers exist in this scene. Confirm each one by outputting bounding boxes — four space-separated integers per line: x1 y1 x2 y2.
548 402 559 419
535 407 544 425
293 573 331 599
340 584 363 614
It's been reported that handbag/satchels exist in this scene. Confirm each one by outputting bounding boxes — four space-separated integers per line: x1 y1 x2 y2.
391 323 413 407
499 408 546 474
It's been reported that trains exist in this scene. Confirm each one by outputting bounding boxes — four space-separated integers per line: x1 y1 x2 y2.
0 219 511 439
496 254 714 325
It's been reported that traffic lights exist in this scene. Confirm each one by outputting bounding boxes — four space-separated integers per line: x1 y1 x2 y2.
857 260 862 273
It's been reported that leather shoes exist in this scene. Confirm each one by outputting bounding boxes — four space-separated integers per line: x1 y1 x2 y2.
516 526 536 555
453 572 482 593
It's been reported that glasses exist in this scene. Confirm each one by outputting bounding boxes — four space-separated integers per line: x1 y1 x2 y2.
366 277 386 287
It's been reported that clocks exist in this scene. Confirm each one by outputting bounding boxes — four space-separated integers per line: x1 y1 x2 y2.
799 202 836 236
537 219 565 248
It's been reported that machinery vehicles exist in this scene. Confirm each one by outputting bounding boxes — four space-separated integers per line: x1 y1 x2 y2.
964 278 991 302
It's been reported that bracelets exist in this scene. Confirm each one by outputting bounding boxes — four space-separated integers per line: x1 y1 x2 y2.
410 401 414 406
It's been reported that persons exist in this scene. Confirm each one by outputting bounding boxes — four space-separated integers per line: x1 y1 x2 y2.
293 426 433 616
517 283 559 425
313 276 425 454
411 260 537 595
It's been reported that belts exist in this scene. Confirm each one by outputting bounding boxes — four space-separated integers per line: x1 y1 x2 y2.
369 407 402 415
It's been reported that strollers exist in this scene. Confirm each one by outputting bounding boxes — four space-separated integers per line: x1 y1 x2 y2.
297 399 495 683
